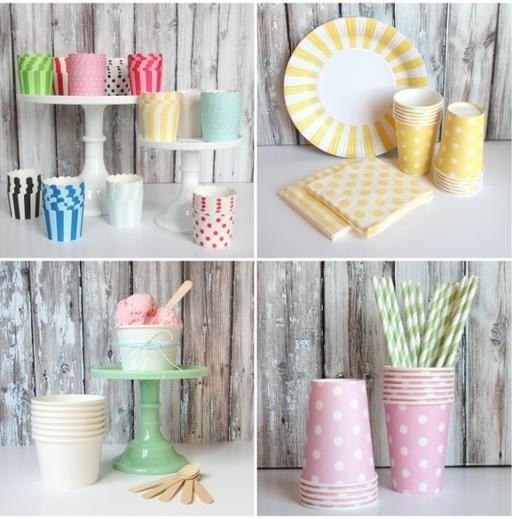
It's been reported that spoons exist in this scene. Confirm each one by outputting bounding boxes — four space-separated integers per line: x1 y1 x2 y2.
127 461 216 506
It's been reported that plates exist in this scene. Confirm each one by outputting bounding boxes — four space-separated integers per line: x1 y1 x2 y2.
282 18 429 159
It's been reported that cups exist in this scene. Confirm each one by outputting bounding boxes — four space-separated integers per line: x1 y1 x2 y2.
391 87 444 178
28 393 108 488
7 162 240 252
115 323 182 371
138 89 241 141
379 365 457 497
298 377 379 509
16 51 164 96
432 101 486 197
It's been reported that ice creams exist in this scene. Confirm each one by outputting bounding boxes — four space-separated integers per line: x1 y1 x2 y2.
115 294 182 326
